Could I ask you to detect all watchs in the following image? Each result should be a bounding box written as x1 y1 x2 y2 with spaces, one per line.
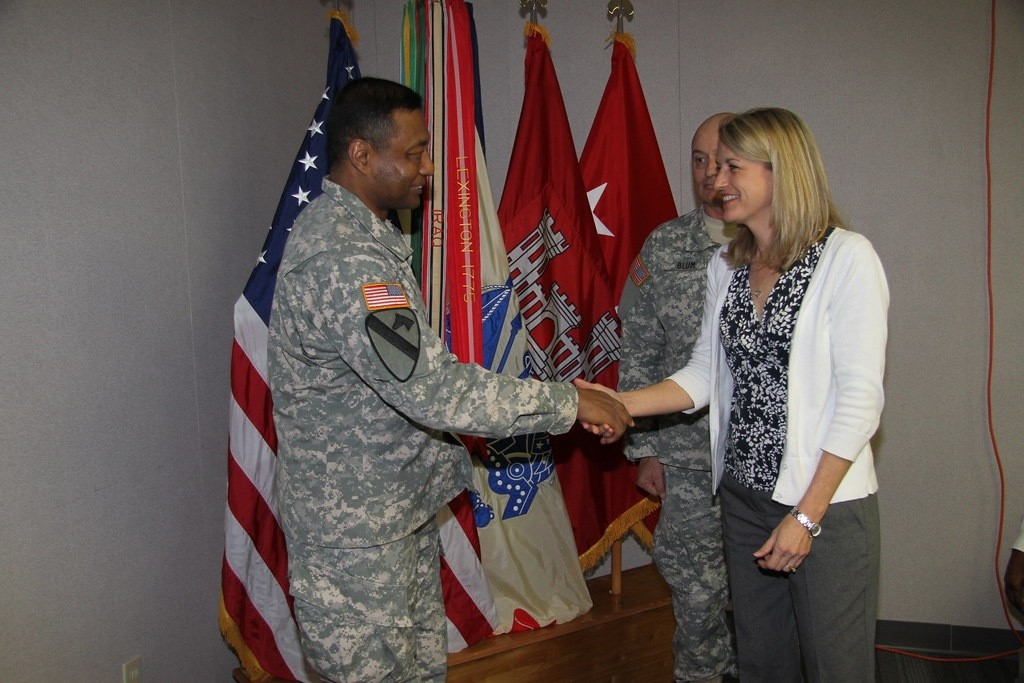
789 503 823 539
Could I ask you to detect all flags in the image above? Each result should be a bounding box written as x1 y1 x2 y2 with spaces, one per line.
215 0 494 683
402 0 592 633
491 19 682 571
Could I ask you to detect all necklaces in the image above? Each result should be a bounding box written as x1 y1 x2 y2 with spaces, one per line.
750 263 778 297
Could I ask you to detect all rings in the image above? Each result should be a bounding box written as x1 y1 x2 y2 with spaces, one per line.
786 562 796 572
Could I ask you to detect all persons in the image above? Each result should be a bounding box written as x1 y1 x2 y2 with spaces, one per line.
569 108 891 683
618 110 738 683
269 74 643 683
